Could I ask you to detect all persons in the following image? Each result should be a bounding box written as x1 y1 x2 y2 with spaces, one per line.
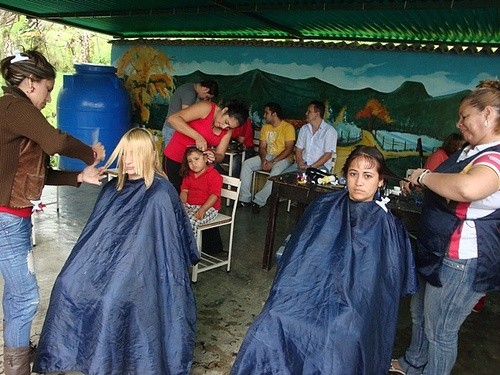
32 128 203 375
236 102 296 207
386 80 500 375
0 49 109 375
280 100 336 175
226 145 417 375
160 80 253 260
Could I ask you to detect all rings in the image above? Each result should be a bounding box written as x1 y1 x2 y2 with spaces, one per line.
401 185 405 189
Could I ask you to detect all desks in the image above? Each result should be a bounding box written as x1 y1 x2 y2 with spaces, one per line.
263 171 423 272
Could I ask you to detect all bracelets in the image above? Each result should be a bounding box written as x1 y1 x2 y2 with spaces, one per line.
418 168 431 188
271 159 275 164
92 148 98 161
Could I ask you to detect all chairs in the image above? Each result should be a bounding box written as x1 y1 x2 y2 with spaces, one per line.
189 150 292 283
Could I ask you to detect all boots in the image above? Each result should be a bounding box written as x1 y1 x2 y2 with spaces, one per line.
3 345 30 375
30 340 37 362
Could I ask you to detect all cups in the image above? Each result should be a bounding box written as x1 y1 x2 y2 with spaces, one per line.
394 186 401 196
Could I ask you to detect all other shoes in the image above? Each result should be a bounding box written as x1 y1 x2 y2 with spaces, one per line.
253 203 260 215
388 359 407 375
237 201 250 208
